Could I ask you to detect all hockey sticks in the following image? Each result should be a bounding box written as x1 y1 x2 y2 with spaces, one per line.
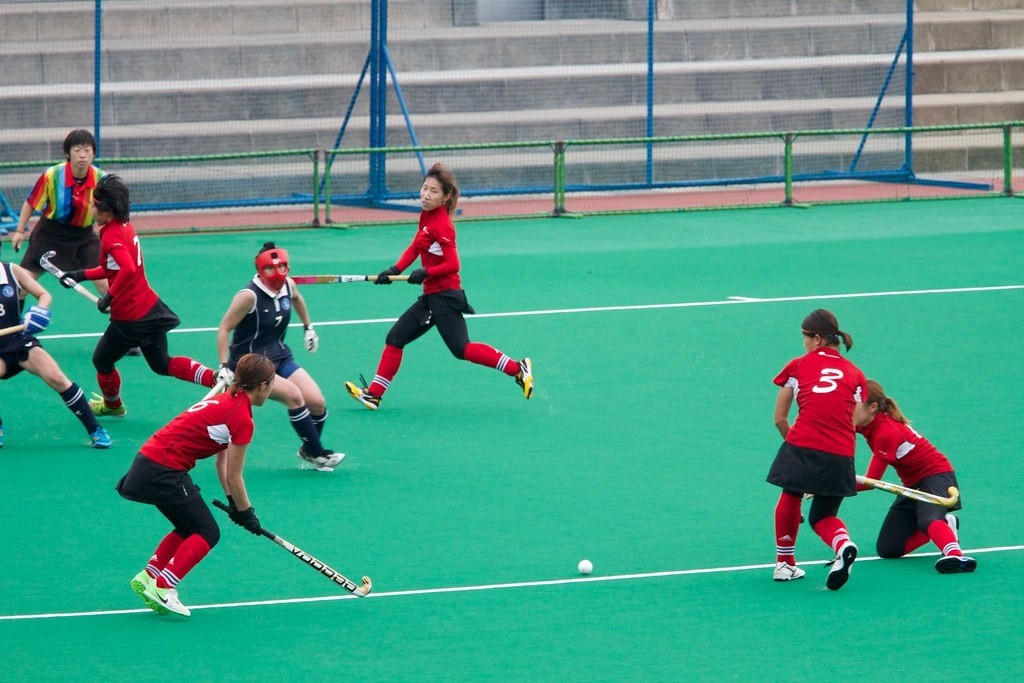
856 474 961 508
0 324 26 337
211 497 373 599
291 274 412 285
38 250 112 312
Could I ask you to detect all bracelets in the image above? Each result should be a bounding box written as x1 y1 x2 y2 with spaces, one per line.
16 230 25 234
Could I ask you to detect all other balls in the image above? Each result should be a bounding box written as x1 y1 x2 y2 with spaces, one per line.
578 559 593 574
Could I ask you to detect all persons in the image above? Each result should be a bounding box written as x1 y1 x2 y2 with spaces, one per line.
344 162 533 411
12 129 141 356
217 241 346 472
115 353 275 617
852 379 977 574
766 308 868 591
0 241 113 450
60 174 220 418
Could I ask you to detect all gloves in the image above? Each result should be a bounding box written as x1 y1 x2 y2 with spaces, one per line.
21 306 51 339
226 495 240 524
240 507 261 537
96 291 112 314
305 329 318 354
408 268 426 285
217 368 234 385
374 266 400 284
59 270 85 288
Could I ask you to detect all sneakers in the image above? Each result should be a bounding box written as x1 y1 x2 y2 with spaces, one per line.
89 391 126 418
127 347 141 356
1 426 4 446
345 374 380 411
942 514 960 543
311 452 346 467
89 426 113 448
773 561 805 581
515 358 533 398
214 367 228 395
296 447 316 463
131 570 170 616
143 579 191 617
826 542 858 591
935 556 977 574
